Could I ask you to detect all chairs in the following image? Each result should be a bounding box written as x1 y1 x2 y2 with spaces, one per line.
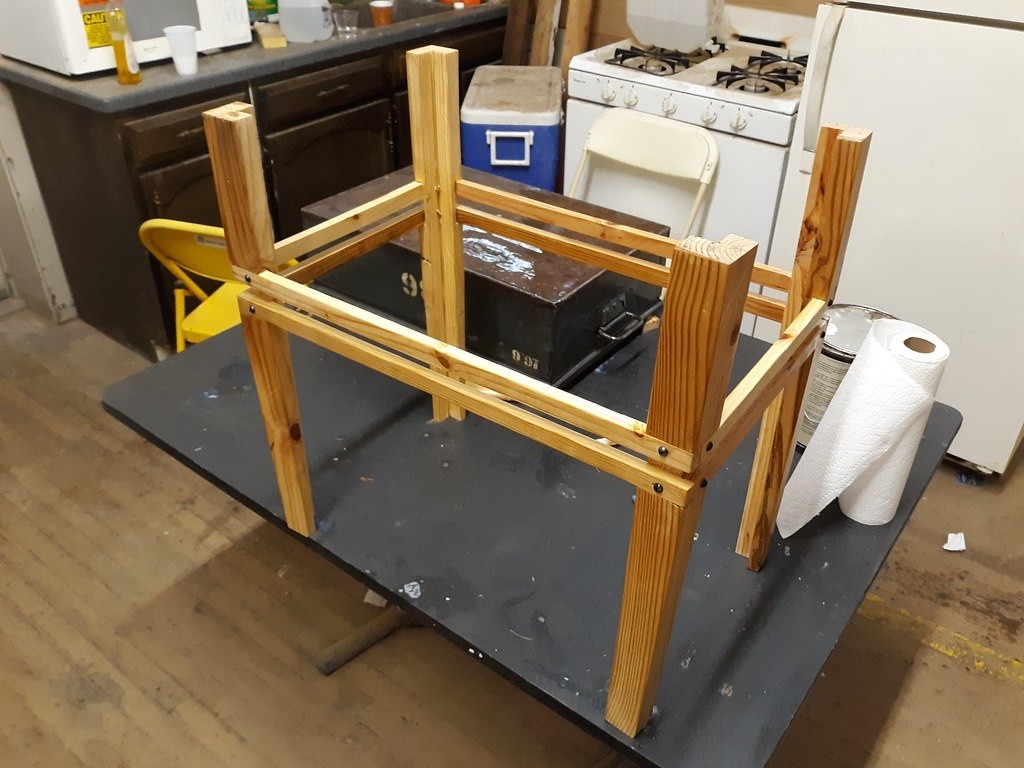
141 214 313 354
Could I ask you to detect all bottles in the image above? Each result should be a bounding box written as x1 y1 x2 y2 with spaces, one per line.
103 0 142 87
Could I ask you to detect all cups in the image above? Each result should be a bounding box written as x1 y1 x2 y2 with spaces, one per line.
331 10 359 39
368 0 394 28
163 25 197 76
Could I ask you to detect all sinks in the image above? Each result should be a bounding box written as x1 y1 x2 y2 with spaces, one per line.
325 0 458 34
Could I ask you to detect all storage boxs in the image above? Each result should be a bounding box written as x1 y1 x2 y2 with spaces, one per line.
459 61 566 197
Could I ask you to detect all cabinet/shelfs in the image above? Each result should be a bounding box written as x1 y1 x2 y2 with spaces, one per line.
0 0 513 366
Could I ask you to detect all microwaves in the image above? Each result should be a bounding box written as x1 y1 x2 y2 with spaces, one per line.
1 0 255 79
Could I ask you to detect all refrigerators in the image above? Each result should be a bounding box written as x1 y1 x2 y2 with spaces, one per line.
752 2 1024 486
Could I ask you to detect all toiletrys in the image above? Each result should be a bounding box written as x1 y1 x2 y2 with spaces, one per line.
103 1 145 86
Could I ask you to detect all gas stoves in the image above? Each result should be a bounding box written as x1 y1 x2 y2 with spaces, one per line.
604 36 808 98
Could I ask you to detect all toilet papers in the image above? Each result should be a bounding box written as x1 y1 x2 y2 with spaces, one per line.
773 316 951 542
